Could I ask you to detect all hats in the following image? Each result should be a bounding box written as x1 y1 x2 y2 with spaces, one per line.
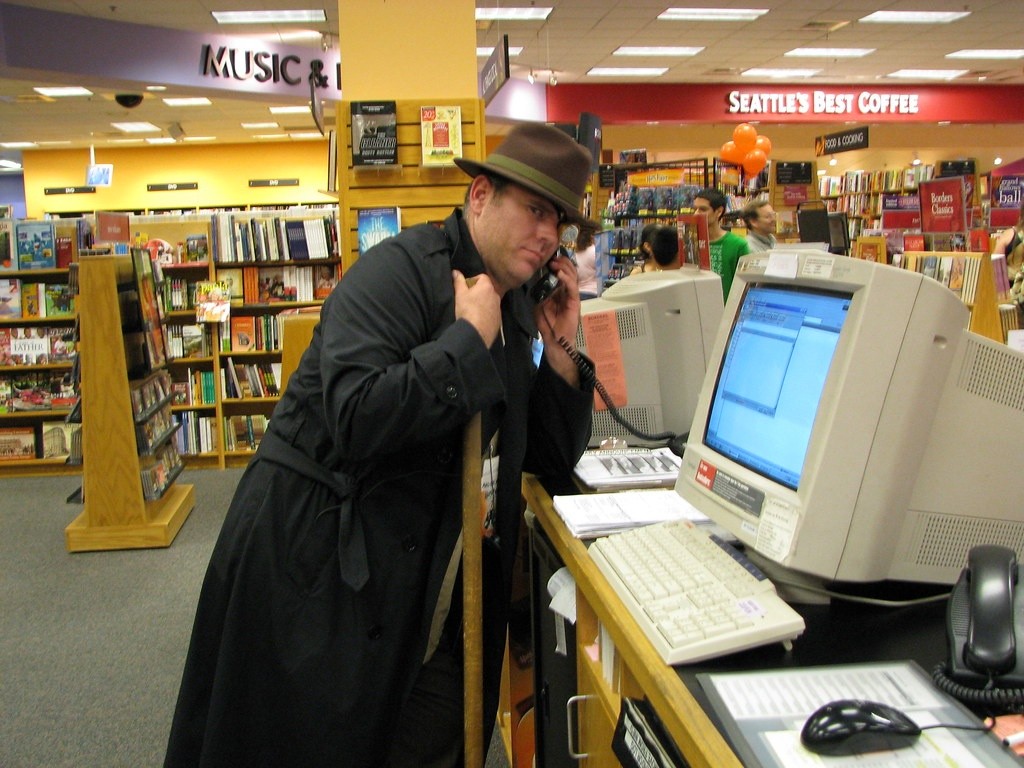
452 122 603 232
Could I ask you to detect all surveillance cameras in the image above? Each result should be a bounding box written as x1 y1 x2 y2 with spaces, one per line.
114 91 143 108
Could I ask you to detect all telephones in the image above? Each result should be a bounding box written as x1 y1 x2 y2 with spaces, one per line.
531 245 570 306
944 545 1024 684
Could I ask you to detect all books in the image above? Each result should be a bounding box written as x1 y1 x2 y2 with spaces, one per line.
211 203 336 263
0 277 75 318
0 369 78 413
171 359 285 405
168 309 285 357
132 370 180 499
173 412 272 456
0 420 83 466
0 327 75 365
165 263 344 310
818 164 1018 346
0 203 132 273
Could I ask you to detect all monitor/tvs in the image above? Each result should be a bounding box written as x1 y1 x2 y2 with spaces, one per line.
673 249 1024 605
565 264 725 447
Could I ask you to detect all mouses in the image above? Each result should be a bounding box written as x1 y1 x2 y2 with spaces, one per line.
801 699 921 757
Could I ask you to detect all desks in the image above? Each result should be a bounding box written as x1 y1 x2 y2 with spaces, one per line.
492 445 1024 768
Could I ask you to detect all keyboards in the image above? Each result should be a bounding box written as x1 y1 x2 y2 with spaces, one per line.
588 514 806 668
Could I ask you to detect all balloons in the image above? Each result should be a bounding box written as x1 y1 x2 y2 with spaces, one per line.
721 124 771 178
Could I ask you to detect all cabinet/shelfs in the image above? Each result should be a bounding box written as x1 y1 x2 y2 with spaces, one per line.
1 186 1024 554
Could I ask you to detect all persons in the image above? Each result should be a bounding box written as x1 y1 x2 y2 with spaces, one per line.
993 195 1024 319
54 335 67 355
15 355 21 364
631 221 685 276
571 222 600 300
11 327 18 338
37 328 45 338
162 119 597 768
38 354 48 363
24 328 31 338
690 187 749 304
740 200 779 255
